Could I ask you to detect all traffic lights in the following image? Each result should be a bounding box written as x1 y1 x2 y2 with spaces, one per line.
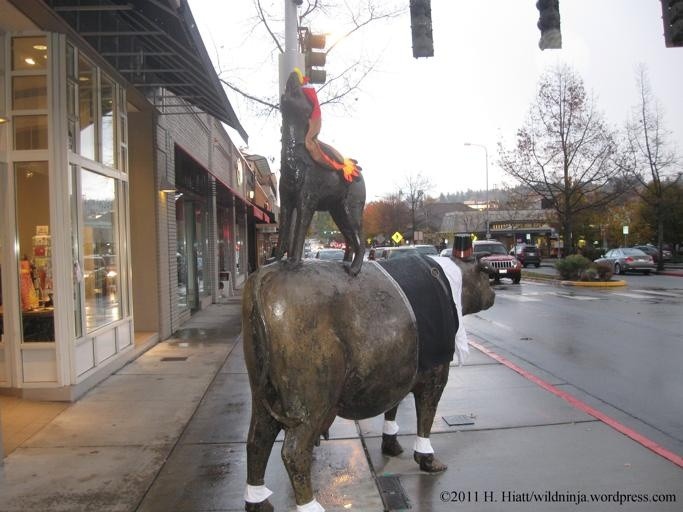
297 29 327 87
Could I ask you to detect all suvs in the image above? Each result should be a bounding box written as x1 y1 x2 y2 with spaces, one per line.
471 240 521 285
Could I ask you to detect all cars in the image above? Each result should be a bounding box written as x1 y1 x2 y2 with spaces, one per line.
510 244 541 267
591 244 672 273
306 246 452 268
84 254 119 297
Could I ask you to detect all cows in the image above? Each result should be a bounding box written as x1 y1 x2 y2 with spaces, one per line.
241 248 497 512
